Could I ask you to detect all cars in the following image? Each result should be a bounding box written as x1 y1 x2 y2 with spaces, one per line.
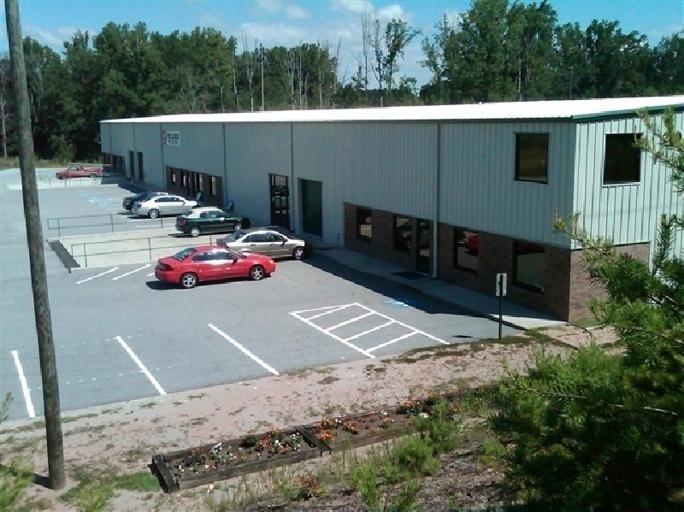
131 195 197 219
465 231 546 254
123 190 169 214
216 228 309 261
155 246 275 288
175 208 251 237
396 219 466 249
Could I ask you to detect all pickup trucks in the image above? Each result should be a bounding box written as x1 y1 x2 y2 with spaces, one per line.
56 164 101 179
